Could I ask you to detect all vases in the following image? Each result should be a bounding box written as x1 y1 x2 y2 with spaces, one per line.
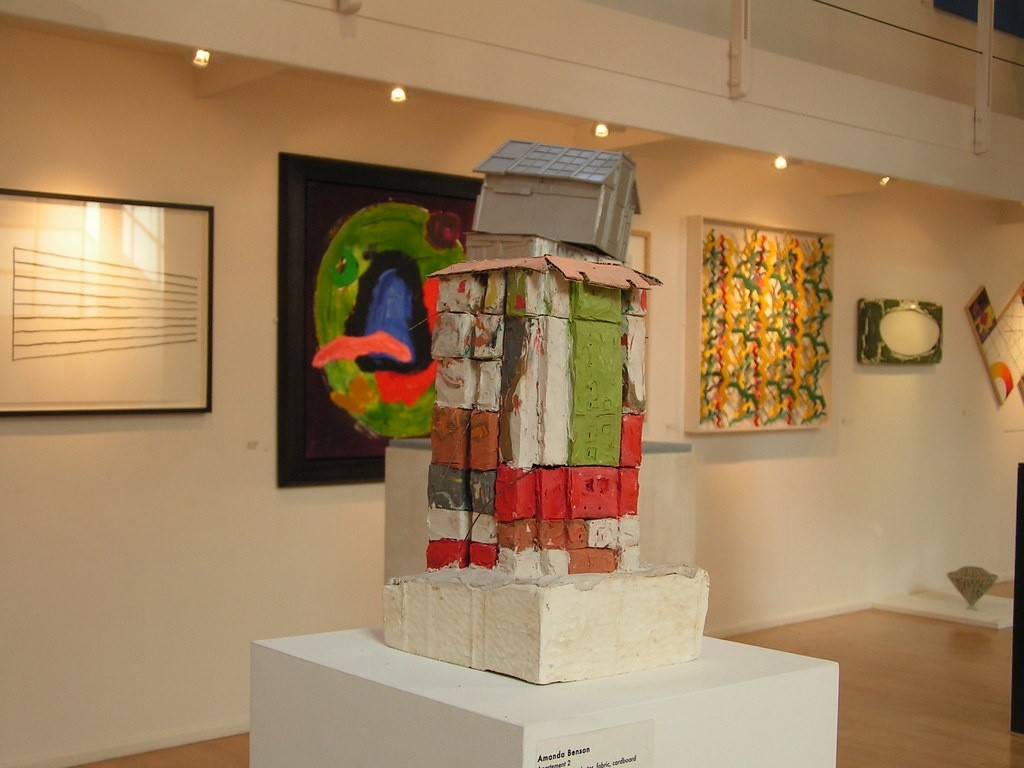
274 151 484 488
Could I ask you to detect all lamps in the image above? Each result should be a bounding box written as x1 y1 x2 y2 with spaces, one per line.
858 292 943 366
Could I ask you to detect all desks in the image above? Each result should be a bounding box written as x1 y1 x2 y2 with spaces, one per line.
247 628 841 768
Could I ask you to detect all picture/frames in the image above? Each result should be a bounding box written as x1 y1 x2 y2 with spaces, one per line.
686 211 834 441
625 231 651 278
965 280 1024 404
2 187 216 417
